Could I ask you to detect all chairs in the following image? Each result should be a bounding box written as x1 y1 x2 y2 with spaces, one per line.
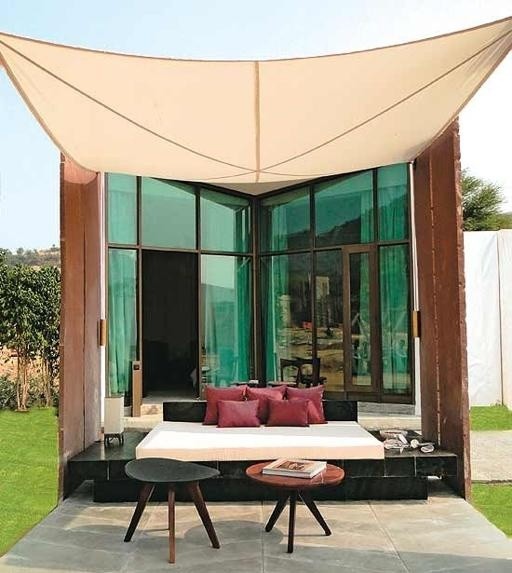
280 356 327 385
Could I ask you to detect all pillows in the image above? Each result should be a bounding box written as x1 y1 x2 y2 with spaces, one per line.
202 384 328 428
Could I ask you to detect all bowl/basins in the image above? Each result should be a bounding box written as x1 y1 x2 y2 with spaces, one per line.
379 429 408 440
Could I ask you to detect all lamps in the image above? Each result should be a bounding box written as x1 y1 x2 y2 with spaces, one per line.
103 395 126 448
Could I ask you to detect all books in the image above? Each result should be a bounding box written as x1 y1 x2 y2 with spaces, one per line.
261 457 328 480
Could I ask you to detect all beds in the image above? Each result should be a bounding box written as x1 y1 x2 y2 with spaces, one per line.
134 400 385 462
